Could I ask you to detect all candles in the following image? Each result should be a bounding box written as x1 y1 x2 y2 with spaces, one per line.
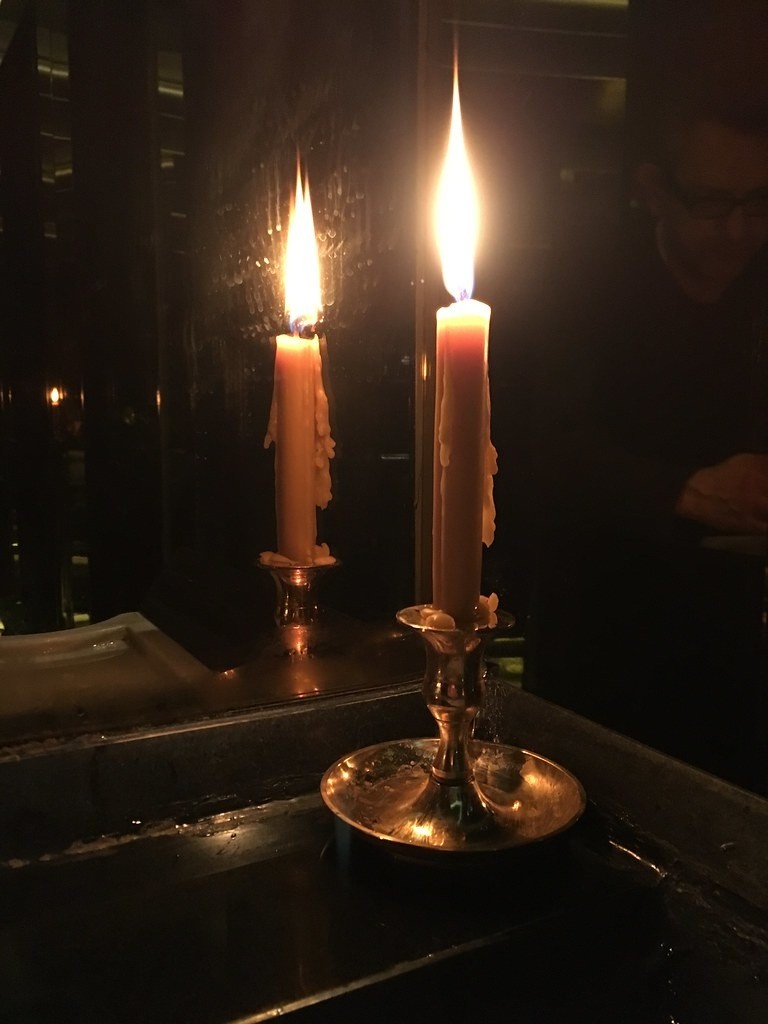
430 37 500 614
264 139 337 560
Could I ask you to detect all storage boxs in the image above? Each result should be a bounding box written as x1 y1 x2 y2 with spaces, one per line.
0 676 768 1024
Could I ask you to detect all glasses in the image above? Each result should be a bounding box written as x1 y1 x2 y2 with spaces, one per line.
654 159 768 222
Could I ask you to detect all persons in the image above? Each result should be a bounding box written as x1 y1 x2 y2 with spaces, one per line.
530 80 768 791
